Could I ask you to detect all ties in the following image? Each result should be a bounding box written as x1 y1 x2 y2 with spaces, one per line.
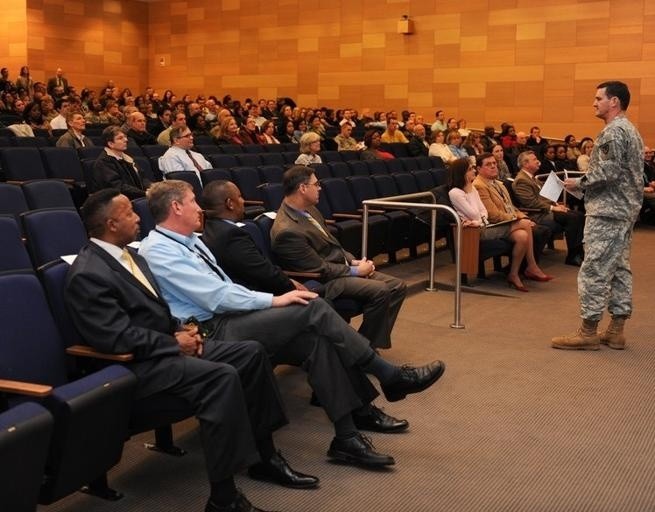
122 251 158 298
186 149 203 172
307 213 329 237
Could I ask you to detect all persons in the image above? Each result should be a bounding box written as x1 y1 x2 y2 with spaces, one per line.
3 63 591 193
63 165 444 512
448 150 592 293
643 144 655 207
551 80 645 352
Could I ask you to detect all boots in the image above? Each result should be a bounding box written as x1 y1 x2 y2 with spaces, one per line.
551 319 600 350
598 317 625 349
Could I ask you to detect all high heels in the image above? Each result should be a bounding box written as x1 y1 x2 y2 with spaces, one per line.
524 270 554 281
507 275 528 292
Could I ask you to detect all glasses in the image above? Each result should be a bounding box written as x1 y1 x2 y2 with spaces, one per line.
306 181 320 186
176 133 193 138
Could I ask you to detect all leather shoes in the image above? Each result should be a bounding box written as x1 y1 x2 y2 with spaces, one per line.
327 432 394 465
355 402 409 432
247 449 319 488
204 488 264 512
380 360 445 402
565 256 583 266
